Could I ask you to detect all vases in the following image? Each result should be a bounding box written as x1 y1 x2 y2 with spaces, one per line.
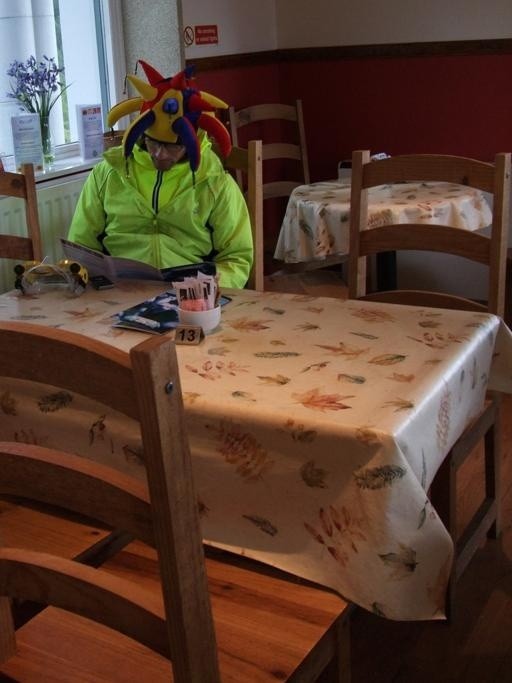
36 111 55 169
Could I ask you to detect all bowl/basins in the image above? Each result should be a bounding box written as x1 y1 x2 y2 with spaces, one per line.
177 305 222 334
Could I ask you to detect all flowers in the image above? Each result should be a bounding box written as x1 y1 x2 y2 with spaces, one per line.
7 54 78 153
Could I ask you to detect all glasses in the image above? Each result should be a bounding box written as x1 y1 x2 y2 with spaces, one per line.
142 139 183 153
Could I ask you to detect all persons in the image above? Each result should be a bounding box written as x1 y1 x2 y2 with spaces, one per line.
64 59 253 288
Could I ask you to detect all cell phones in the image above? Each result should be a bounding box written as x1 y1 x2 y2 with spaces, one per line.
89 274 114 291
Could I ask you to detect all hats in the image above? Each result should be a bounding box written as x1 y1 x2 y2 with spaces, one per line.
106 59 232 189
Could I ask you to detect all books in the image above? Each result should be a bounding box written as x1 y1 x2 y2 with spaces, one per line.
57 236 218 281
107 285 234 336
